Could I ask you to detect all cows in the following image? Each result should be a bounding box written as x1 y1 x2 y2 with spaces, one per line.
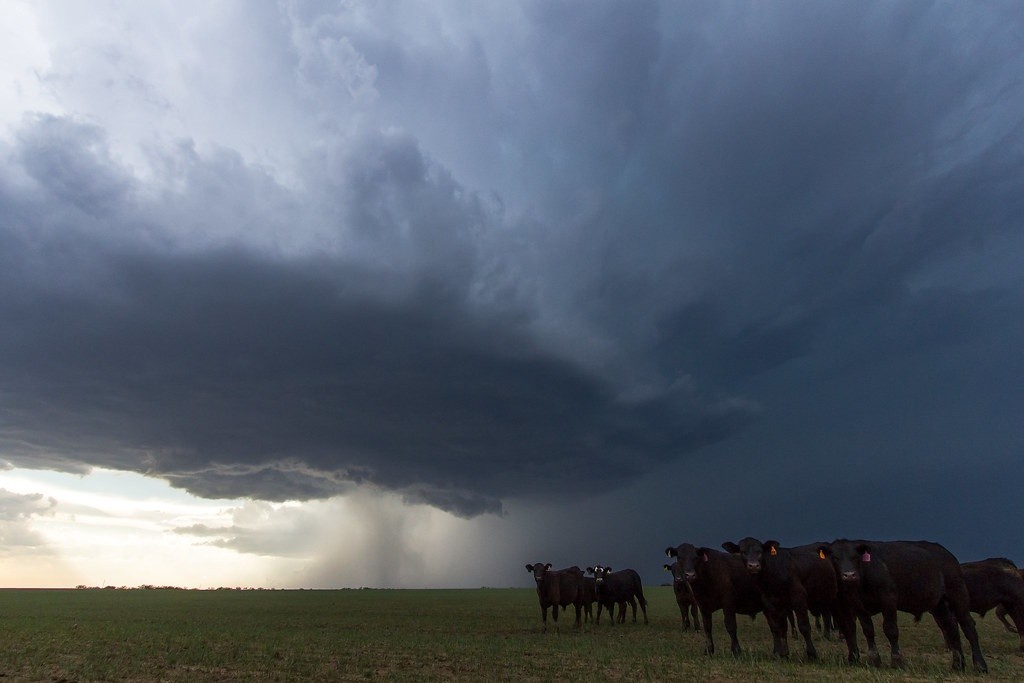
660 537 1024 674
524 562 651 628
